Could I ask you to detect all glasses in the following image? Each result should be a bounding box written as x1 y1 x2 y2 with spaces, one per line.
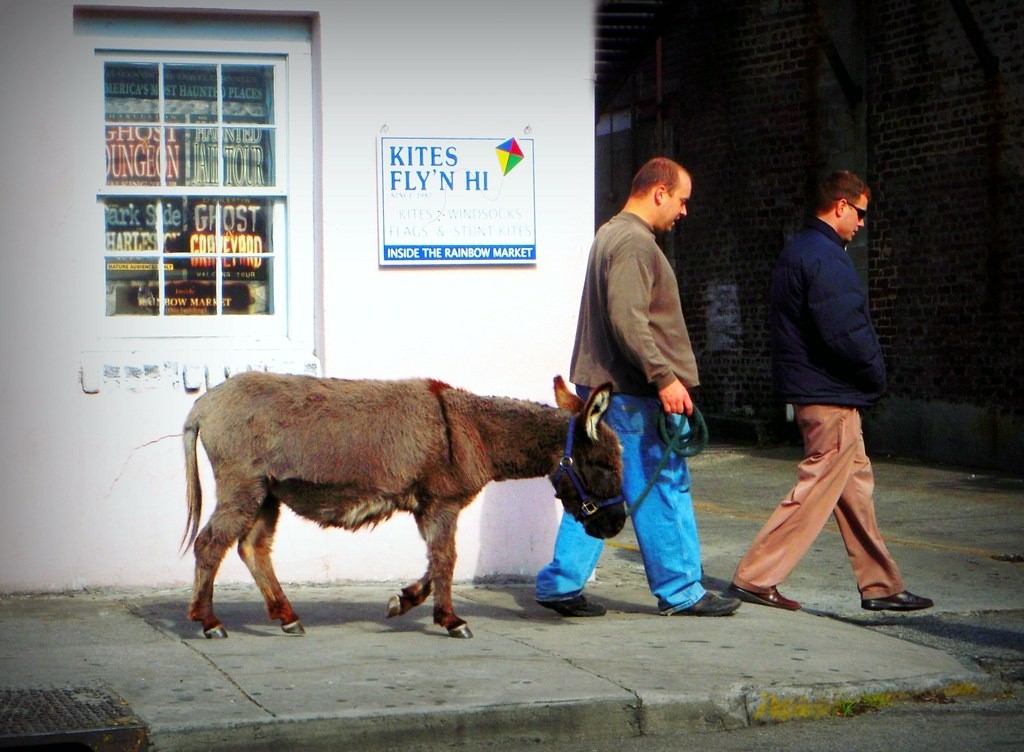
836 196 867 219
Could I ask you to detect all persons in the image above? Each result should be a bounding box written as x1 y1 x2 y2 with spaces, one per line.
534 155 742 619
727 168 936 613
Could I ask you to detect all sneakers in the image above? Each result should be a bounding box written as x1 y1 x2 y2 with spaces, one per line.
536 593 607 617
674 591 742 615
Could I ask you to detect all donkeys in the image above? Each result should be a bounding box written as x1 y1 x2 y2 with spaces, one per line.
175 371 624 641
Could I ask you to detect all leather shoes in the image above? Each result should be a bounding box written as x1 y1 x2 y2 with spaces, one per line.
859 589 934 611
728 580 802 611
